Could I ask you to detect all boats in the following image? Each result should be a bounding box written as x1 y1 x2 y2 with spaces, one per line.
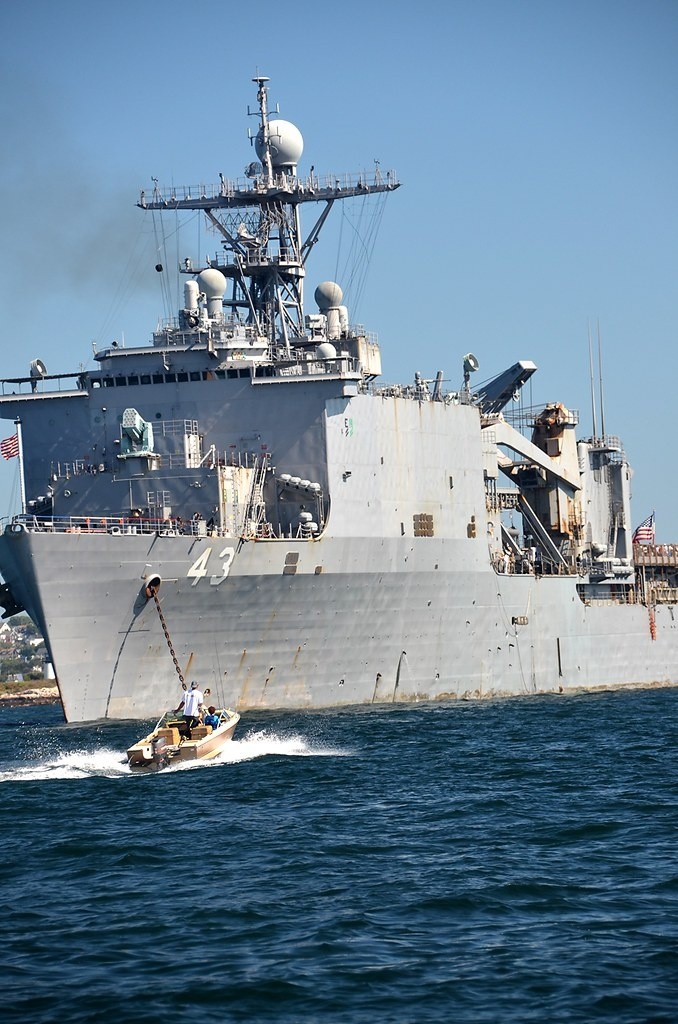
126 702 242 774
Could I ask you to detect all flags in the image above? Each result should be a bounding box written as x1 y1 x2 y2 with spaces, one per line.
629 512 656 548
0 432 21 463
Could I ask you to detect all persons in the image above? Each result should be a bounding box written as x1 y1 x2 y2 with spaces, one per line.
628 571 675 603
201 705 223 729
503 541 511 573
576 553 583 574
172 679 206 744
128 507 218 536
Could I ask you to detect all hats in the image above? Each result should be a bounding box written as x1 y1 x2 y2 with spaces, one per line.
191 680 199 688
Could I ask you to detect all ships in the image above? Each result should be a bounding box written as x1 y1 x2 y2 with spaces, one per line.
1 69 676 719
